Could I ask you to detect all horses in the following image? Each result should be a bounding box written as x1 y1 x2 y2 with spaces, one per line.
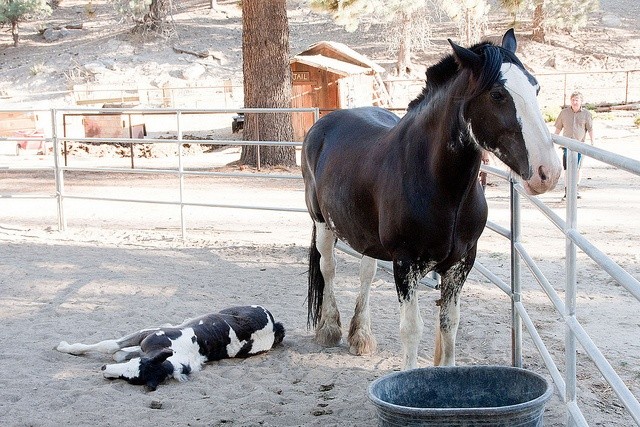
300 27 562 373
57 305 286 394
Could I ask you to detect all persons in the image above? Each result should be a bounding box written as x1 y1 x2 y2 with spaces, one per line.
553 91 594 200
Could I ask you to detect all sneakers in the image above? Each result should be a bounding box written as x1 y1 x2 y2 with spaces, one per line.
562 194 566 201
577 191 581 199
